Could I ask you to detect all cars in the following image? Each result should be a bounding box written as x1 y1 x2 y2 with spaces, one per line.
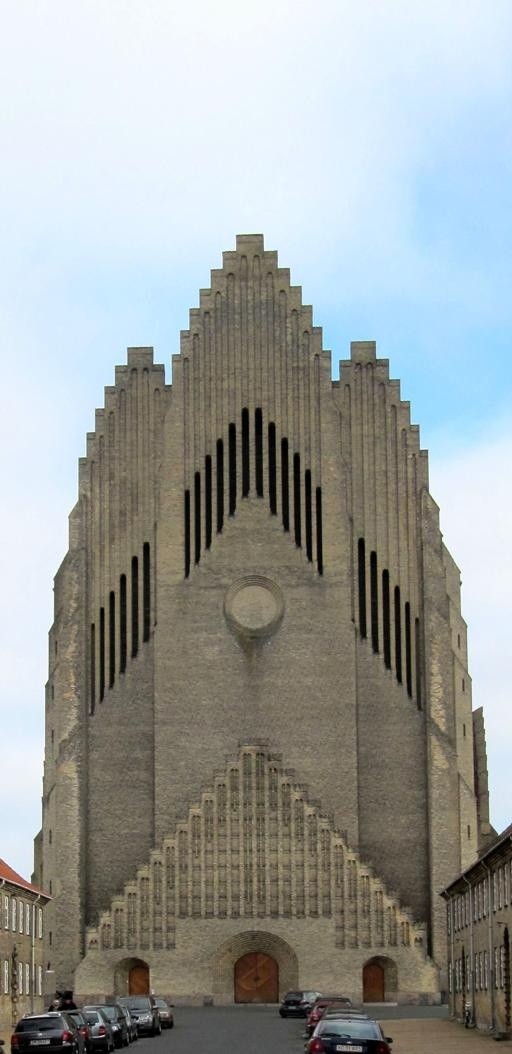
11 995 174 1054
280 990 393 1054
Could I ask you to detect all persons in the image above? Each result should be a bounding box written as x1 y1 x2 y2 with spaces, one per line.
48 996 77 1011
464 1008 471 1028
